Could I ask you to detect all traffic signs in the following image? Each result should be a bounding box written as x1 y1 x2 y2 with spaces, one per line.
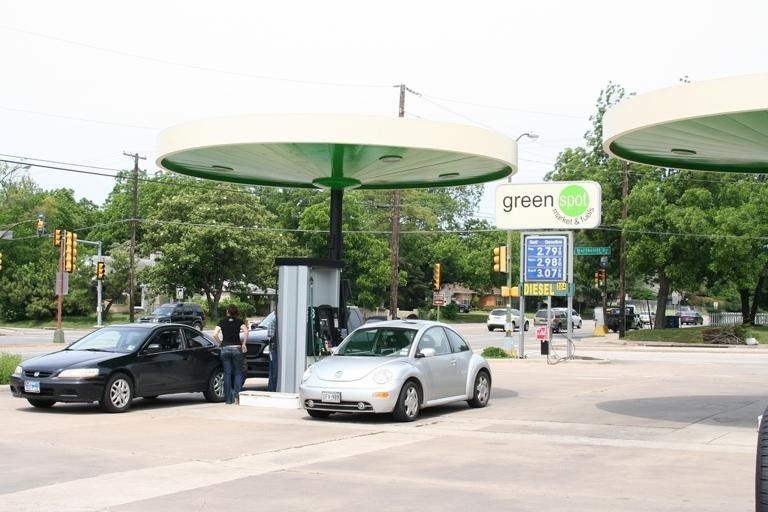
574 246 611 255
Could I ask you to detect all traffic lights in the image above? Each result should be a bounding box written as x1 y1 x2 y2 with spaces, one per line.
38 220 46 232
98 262 105 279
54 230 62 246
599 268 607 287
500 285 509 297
433 263 441 291
64 231 77 272
493 245 507 273
595 273 599 288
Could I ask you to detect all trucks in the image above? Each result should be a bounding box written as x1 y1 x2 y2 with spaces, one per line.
600 304 644 331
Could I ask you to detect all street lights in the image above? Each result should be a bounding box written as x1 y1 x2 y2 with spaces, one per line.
0 164 32 182
504 130 539 338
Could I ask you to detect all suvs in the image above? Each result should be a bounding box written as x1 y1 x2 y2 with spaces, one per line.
139 303 205 330
236 304 365 379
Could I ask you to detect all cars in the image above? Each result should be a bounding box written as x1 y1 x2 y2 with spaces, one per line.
680 312 703 325
639 311 656 324
553 306 584 330
486 308 530 332
676 306 694 316
451 298 470 313
299 319 494 420
9 321 249 412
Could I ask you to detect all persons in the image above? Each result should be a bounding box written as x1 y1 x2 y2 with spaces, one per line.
213 304 248 403
266 307 277 392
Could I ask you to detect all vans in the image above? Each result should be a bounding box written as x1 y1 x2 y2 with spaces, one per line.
534 309 574 332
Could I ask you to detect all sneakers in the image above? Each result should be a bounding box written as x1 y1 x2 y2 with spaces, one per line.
234 395 239 404
